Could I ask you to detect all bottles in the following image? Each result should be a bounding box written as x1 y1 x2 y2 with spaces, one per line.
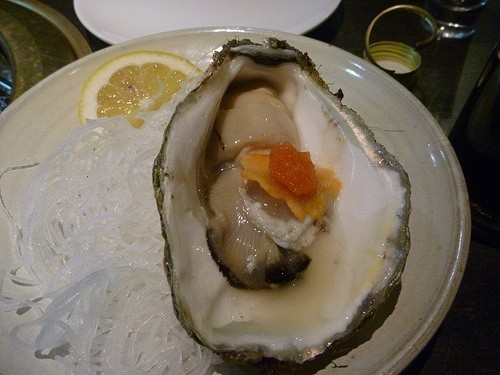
445 37 499 248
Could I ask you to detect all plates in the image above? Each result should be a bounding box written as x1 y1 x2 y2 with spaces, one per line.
72 0 342 47
1 26 472 374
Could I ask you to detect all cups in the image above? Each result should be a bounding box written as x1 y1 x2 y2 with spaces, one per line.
428 0 487 50
362 5 438 93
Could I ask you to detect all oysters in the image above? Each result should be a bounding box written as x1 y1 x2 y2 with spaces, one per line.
153 39 414 368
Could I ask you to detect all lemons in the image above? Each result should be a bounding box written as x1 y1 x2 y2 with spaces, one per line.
77 50 205 133
